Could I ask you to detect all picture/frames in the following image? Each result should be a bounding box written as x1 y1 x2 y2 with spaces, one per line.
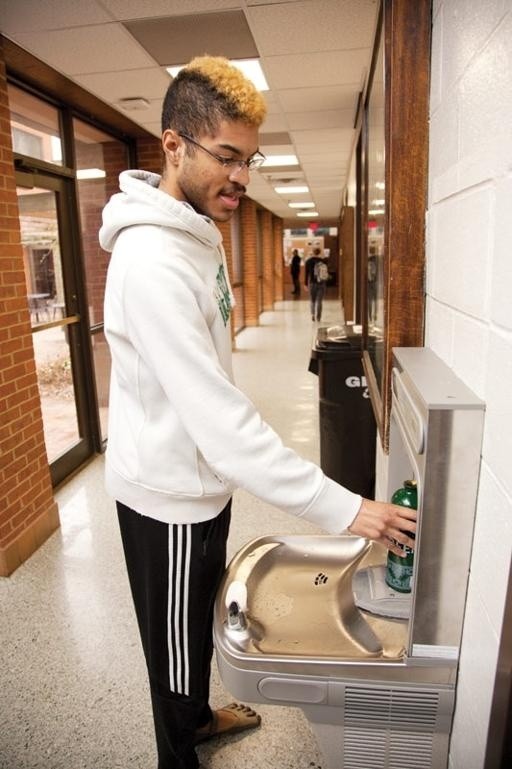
361 0 433 456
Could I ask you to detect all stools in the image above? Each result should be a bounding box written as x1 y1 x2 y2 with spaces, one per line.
53 304 64 320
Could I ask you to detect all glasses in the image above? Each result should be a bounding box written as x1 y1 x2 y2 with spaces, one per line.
177 132 266 175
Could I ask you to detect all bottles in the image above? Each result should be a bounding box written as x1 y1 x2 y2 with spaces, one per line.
385 479 419 592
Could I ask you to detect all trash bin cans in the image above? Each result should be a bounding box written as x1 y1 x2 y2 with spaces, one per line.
312 336 378 501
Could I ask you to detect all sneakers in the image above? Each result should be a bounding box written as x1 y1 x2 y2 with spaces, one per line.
312 316 320 322
193 702 261 746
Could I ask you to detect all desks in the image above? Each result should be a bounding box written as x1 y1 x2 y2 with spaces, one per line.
28 293 50 323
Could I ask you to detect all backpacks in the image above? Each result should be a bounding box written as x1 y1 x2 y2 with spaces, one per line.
314 261 332 282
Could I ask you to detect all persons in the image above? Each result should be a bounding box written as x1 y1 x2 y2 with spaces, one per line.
367 246 378 322
98 52 418 769
289 249 302 298
303 248 333 322
